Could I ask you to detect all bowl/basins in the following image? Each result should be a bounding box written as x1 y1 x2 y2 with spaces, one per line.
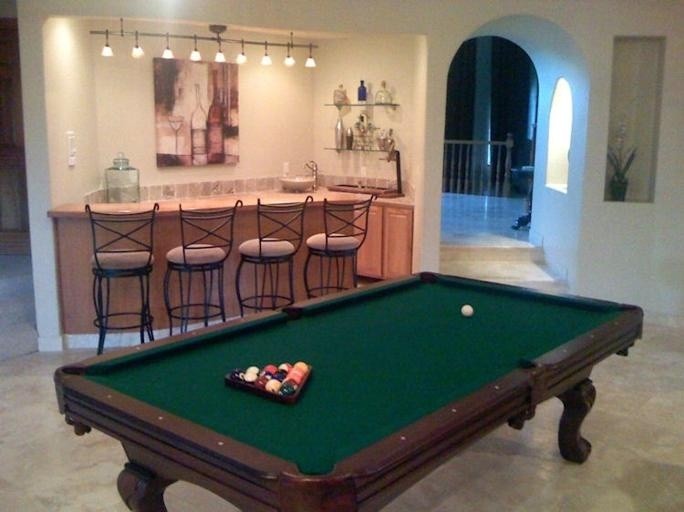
280 177 316 191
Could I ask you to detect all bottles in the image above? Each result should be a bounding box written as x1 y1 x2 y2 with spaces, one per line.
191 85 208 165
335 116 344 150
104 154 140 204
334 82 347 104
208 69 225 163
374 80 392 105
347 127 354 149
358 80 367 104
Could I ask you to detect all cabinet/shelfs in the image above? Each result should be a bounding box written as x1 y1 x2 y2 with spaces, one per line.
354 201 382 280
382 202 412 281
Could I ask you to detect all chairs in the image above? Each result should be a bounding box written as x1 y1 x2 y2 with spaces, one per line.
304 194 378 299
235 196 313 317
85 202 159 356
163 200 243 336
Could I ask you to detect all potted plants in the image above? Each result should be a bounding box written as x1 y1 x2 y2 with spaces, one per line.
607 123 639 202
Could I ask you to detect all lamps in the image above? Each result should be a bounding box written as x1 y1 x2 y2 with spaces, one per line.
215 36 225 62
237 39 247 64
262 41 271 66
190 34 202 61
131 31 143 58
101 28 113 57
305 42 316 68
162 32 174 59
284 42 295 66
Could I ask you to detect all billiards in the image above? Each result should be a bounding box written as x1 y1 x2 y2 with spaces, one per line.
265 379 282 393
258 370 273 380
282 378 298 391
278 384 295 396
461 304 476 318
278 363 293 371
263 364 278 375
230 368 246 382
275 369 288 377
254 377 269 390
291 367 305 378
294 361 308 373
287 373 301 384
244 371 258 382
246 366 259 374
